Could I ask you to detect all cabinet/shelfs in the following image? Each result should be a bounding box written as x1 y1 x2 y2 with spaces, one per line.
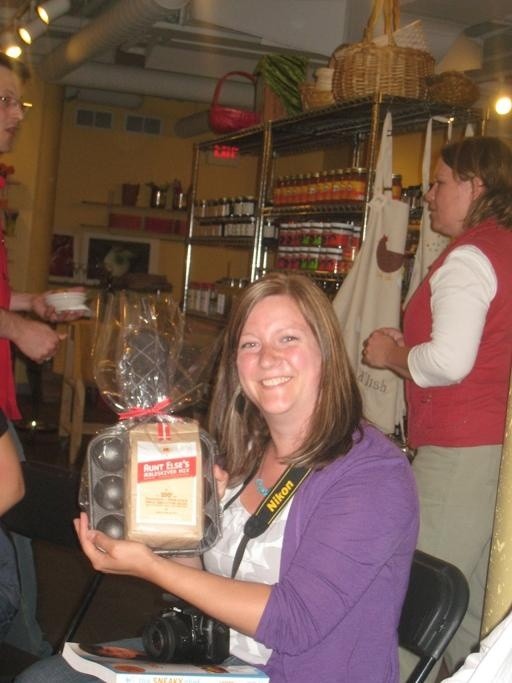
171 94 488 421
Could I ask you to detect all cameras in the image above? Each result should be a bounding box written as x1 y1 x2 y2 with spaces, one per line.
142 603 230 666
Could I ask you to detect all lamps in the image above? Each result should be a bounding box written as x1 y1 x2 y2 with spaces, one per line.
14 1 70 45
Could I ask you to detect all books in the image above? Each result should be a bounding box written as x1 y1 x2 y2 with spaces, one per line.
61 633 270 683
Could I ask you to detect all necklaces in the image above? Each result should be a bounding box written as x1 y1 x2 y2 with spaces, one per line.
249 449 277 501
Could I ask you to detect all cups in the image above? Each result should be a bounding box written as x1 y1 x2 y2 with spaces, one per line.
121 183 139 207
150 189 168 209
172 192 188 210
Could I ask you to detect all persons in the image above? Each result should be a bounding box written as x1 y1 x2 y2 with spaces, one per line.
1 406 28 521
6 268 420 683
358 133 509 683
2 47 89 464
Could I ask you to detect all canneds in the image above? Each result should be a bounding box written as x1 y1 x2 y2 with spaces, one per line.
193 196 278 240
273 169 403 275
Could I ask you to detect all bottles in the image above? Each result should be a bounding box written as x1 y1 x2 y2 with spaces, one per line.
187 274 250 322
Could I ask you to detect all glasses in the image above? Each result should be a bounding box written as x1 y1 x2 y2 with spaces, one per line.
0 97 31 112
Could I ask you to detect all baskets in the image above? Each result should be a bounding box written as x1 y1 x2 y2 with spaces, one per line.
330 0 435 105
209 70 262 135
296 70 480 111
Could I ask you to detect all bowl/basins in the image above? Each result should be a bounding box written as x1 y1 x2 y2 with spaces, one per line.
45 285 91 314
